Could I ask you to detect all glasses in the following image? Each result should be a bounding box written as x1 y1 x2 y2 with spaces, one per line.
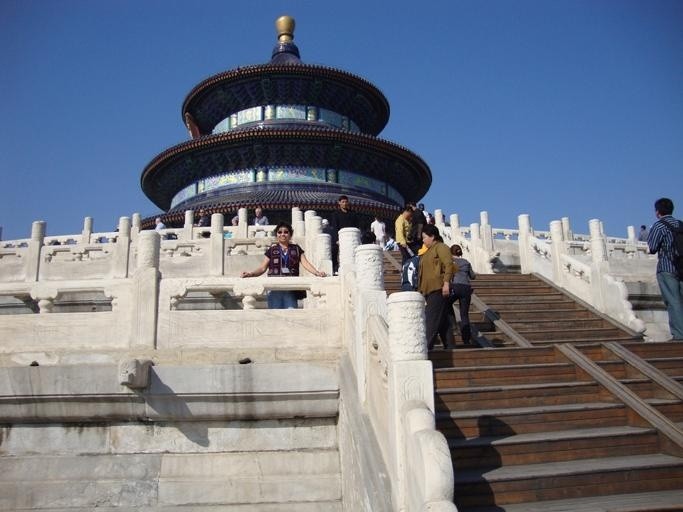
278 230 288 234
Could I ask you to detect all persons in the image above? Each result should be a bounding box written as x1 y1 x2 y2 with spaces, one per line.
193 208 209 238
360 203 444 293
240 221 327 310
329 196 358 277
446 243 475 345
415 223 456 352
153 217 168 240
250 208 269 225
645 197 683 343
636 224 649 242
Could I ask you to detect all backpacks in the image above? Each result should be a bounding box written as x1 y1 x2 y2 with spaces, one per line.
658 218 683 274
401 255 420 290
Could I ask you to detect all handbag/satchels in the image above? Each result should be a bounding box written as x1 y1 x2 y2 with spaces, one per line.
292 290 307 300
